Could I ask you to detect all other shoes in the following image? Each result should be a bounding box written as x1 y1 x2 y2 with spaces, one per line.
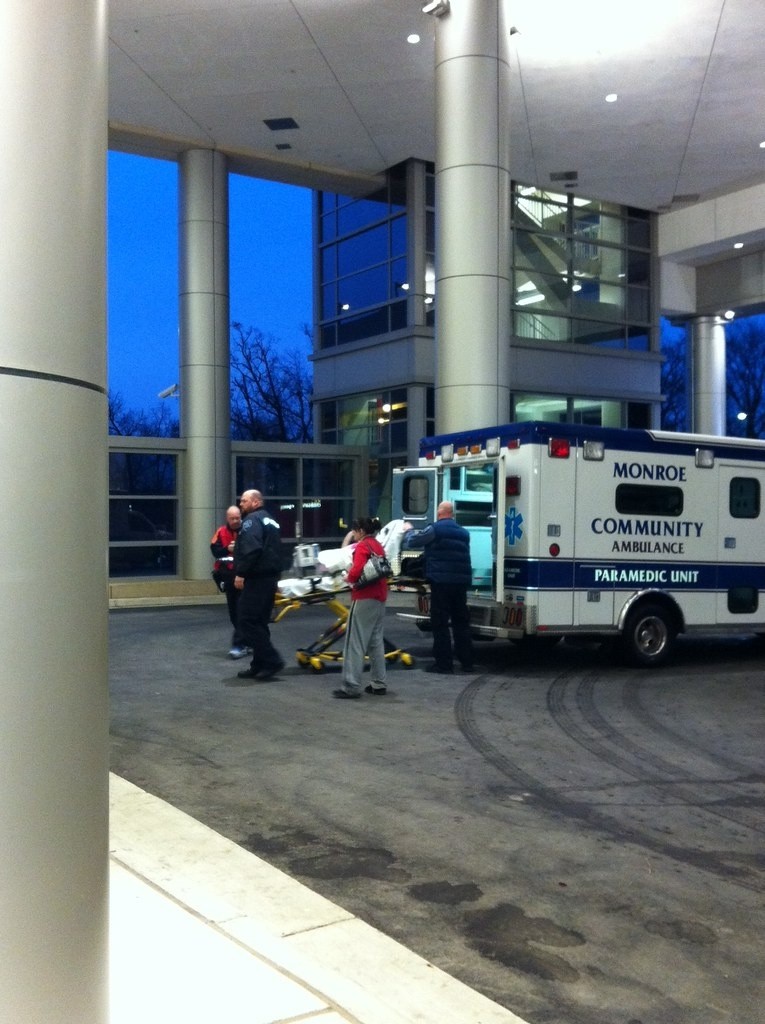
237 644 285 681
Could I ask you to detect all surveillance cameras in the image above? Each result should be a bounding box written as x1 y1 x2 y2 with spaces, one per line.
158 384 177 398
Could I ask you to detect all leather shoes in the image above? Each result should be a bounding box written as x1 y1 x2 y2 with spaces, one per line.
461 662 473 672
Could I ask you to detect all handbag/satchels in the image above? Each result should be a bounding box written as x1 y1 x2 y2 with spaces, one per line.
210 569 226 593
352 540 394 590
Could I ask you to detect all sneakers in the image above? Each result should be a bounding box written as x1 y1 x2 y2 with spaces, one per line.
426 663 454 675
364 684 387 696
332 689 362 699
227 644 254 660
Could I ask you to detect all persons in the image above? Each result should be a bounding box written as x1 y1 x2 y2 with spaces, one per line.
231 490 285 679
404 502 481 672
210 506 253 657
330 517 388 696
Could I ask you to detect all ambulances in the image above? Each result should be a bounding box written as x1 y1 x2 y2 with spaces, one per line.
391 420 765 666
